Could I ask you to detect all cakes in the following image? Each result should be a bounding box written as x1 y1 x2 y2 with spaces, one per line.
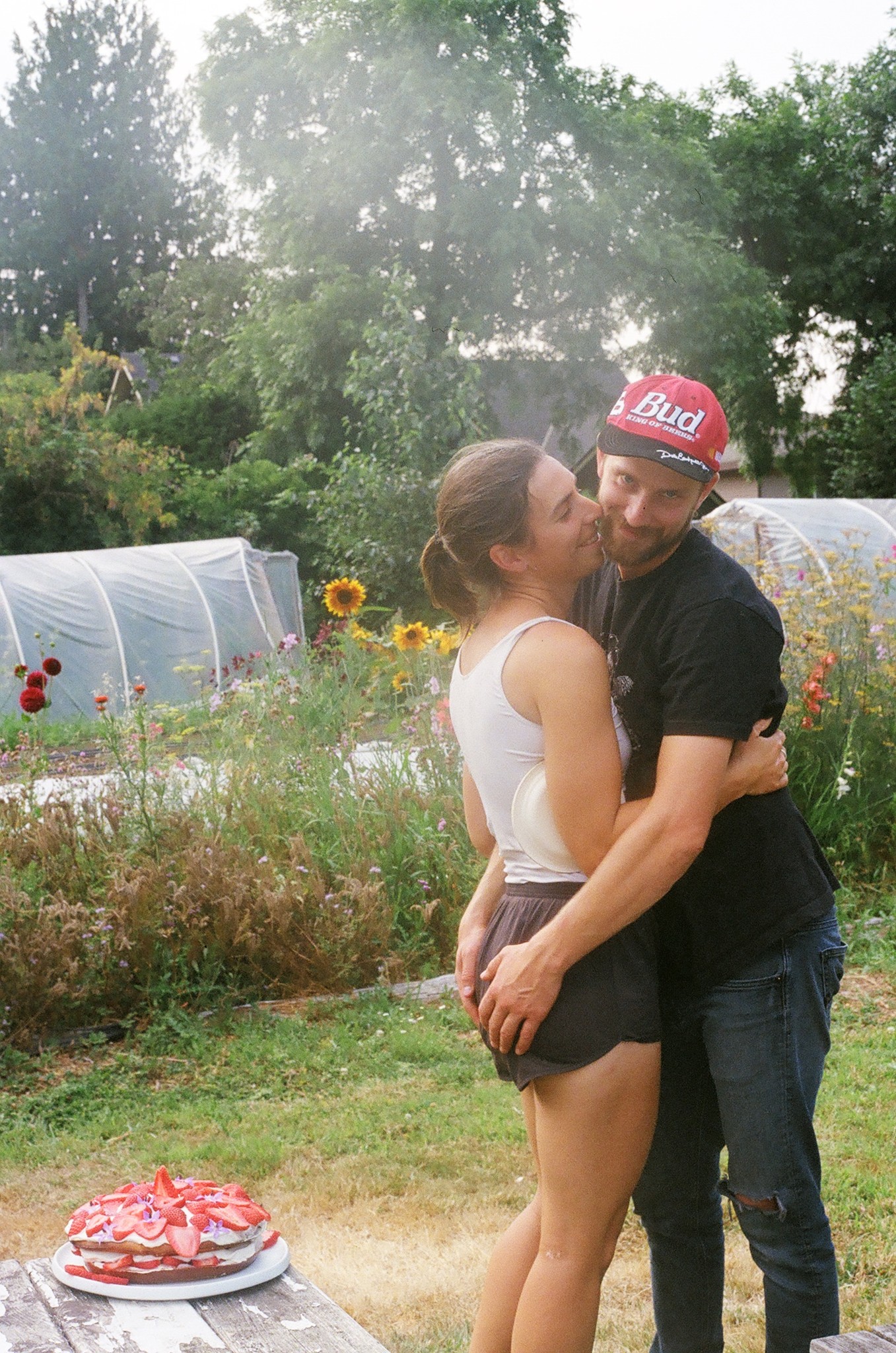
63 1166 281 1283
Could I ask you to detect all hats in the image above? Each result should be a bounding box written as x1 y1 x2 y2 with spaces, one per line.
598 375 728 482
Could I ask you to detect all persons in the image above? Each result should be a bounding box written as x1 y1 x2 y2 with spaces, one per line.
457 375 849 1353
419 439 790 1353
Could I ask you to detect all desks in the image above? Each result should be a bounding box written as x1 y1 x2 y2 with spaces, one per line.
0 1256 391 1353
